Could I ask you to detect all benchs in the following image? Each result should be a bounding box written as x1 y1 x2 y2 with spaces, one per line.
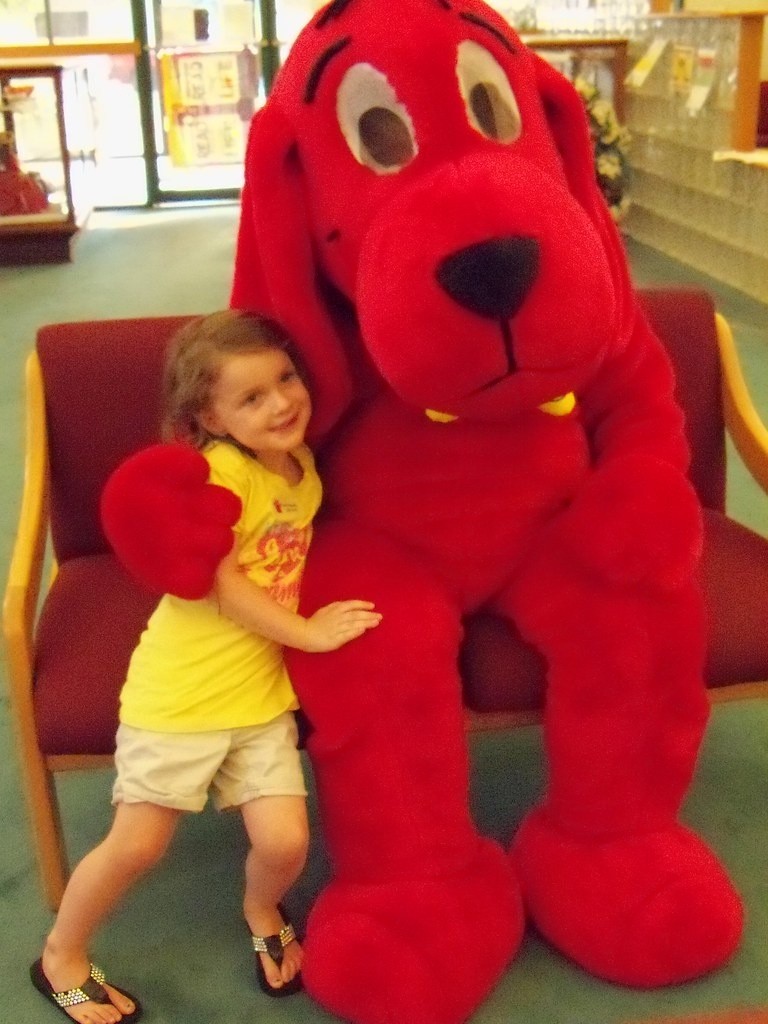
0 286 767 912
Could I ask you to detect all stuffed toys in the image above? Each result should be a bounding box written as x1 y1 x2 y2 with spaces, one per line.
100 2 750 1024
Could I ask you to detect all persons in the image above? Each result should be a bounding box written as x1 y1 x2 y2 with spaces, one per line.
30 302 384 1024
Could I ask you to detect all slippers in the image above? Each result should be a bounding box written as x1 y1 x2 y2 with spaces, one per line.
30 956 144 1024
252 899 307 997
